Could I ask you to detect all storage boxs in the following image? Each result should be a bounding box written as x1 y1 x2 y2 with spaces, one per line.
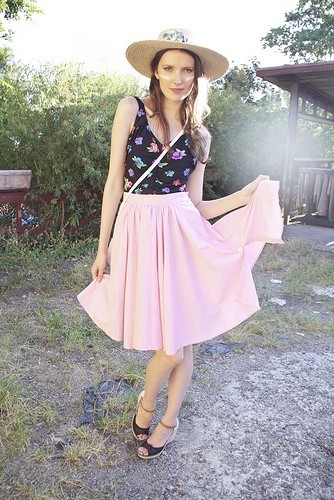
0 169 31 191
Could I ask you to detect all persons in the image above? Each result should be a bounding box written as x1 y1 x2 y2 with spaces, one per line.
76 27 286 460
